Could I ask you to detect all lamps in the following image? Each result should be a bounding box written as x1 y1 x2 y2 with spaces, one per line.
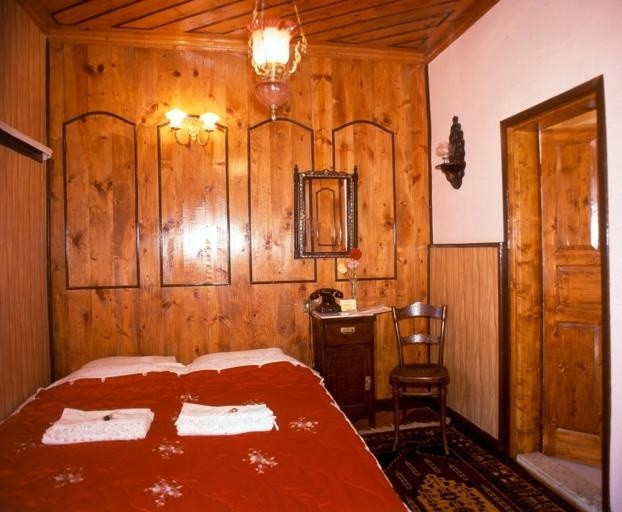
166 108 220 148
434 114 466 191
246 0 309 122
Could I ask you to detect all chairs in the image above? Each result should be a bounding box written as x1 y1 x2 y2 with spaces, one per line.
389 302 449 455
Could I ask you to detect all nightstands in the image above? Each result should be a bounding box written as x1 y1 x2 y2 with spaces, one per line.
309 310 377 429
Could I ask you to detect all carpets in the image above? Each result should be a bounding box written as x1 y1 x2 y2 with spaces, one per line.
356 415 585 511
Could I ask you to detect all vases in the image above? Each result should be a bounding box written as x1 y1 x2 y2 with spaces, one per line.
350 278 362 312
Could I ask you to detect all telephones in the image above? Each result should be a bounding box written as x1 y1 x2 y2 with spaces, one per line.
309 288 343 314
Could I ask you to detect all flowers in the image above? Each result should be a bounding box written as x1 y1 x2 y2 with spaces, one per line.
336 248 362 298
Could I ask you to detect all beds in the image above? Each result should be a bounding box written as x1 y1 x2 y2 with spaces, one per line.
1 348 408 508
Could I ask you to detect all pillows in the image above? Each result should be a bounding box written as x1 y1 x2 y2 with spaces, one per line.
80 347 283 369
